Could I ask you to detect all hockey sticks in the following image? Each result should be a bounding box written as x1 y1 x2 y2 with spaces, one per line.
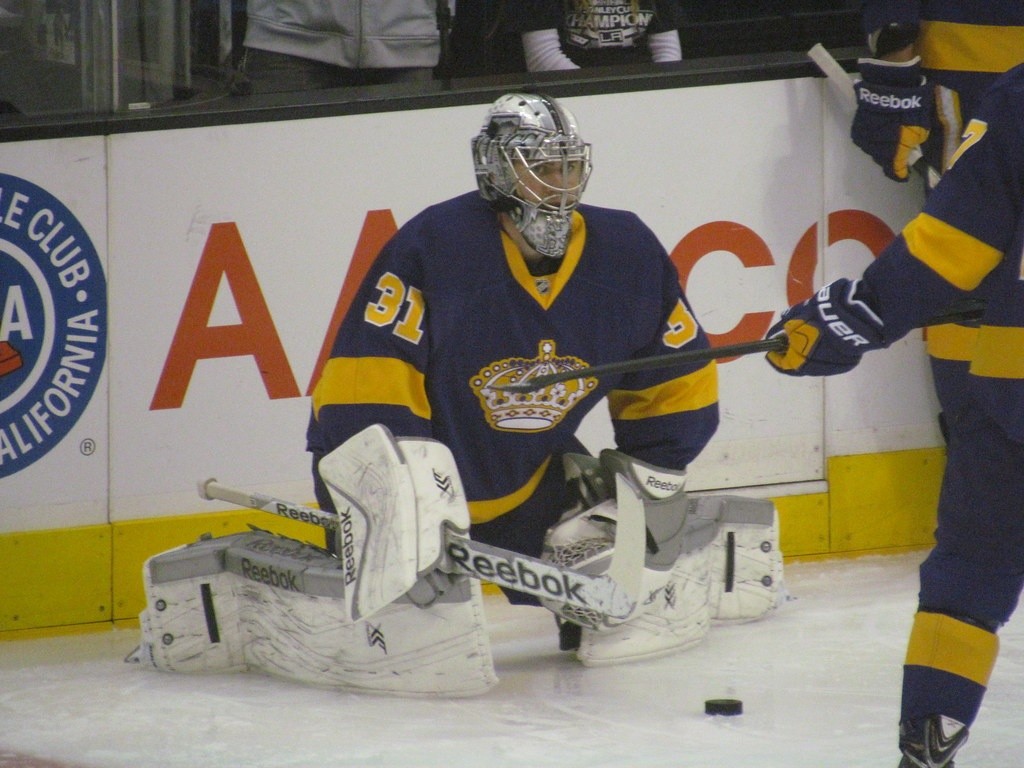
195 474 647 618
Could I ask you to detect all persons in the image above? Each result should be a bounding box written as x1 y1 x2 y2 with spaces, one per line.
241 0 455 90
766 73 1023 768
493 0 683 76
851 0 1024 195
139 91 788 700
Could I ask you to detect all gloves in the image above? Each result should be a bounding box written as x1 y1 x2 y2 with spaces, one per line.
764 272 893 377
542 436 688 634
851 56 936 183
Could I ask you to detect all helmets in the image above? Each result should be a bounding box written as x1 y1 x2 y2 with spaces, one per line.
473 91 592 259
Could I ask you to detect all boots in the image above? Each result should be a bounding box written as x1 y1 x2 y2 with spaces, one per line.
891 714 969 767
